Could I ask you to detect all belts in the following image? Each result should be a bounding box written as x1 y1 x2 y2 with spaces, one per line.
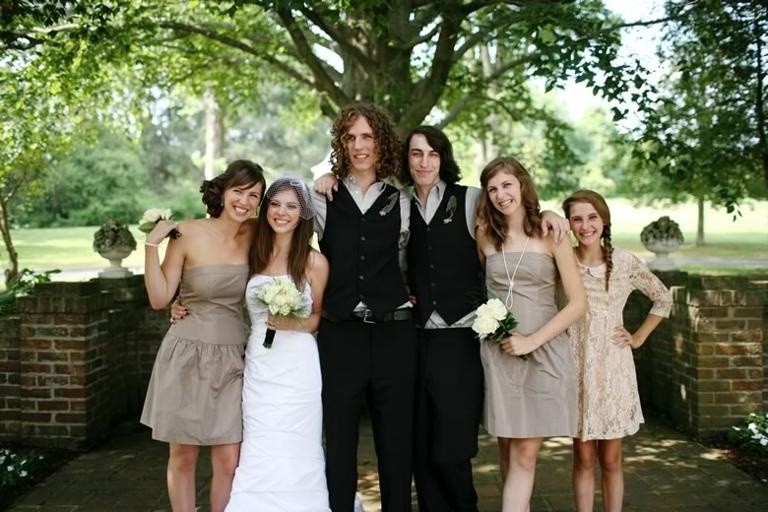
351 309 411 325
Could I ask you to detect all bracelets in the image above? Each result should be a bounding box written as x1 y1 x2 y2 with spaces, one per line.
144 241 160 248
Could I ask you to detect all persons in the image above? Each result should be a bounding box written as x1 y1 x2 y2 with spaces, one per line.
310 126 572 512
168 177 335 512
299 102 419 512
408 156 589 512
556 189 675 512
137 160 266 511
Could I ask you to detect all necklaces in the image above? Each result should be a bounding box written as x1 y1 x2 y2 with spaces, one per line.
498 221 538 310
